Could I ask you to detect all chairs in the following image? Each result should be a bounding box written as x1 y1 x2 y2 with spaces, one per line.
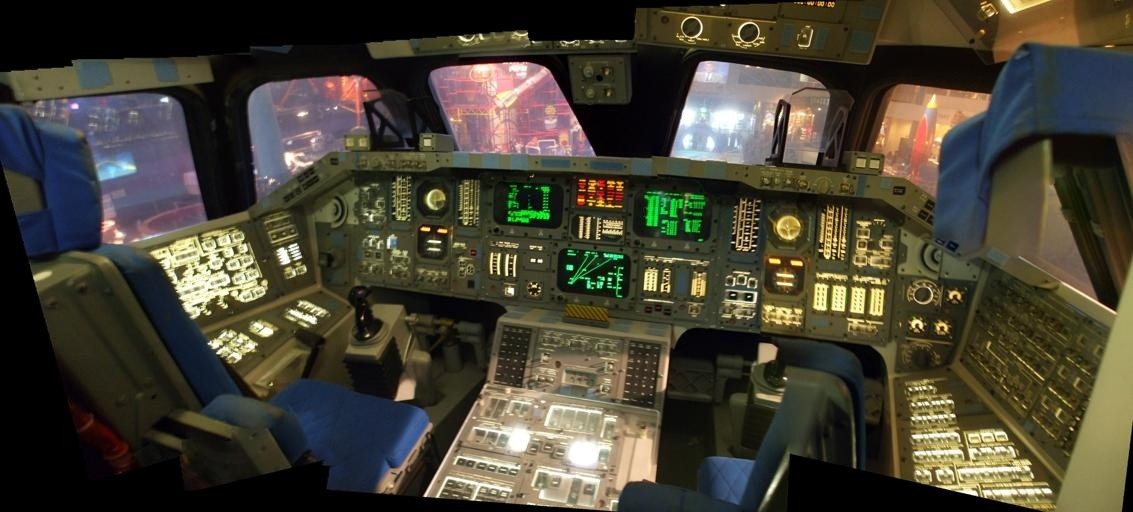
1 100 437 494
618 367 993 512
